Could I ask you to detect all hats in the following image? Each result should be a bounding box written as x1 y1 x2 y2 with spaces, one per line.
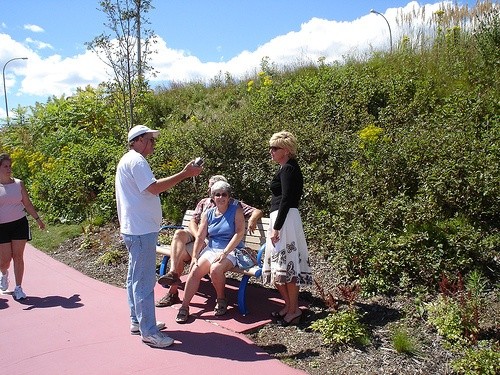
127 125 160 147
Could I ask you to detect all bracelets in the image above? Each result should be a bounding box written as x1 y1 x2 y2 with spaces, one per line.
223 250 228 255
36 217 40 220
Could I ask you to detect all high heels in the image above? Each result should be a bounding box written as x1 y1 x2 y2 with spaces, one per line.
280 314 302 327
271 312 287 320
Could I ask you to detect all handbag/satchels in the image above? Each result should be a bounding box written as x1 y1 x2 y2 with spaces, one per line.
236 247 259 268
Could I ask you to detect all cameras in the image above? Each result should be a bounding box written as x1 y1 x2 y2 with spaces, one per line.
192 157 204 167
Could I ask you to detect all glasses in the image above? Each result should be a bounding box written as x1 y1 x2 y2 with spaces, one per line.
143 138 154 143
216 192 227 197
269 146 283 152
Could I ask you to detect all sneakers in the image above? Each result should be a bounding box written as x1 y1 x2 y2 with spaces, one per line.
130 320 165 335
141 330 174 349
0 270 9 291
14 286 26 300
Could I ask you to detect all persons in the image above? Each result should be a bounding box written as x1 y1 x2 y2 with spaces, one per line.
155 175 263 307
175 181 245 323
115 125 202 346
0 154 44 300
248 132 312 327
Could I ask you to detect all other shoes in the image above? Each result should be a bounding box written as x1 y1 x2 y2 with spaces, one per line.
214 297 230 319
158 270 182 286
175 305 189 324
155 291 180 307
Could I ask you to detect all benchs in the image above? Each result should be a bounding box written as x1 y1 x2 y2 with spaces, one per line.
156 210 270 317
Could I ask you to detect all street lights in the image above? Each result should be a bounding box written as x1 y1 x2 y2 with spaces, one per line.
2 57 28 127
371 9 392 53
